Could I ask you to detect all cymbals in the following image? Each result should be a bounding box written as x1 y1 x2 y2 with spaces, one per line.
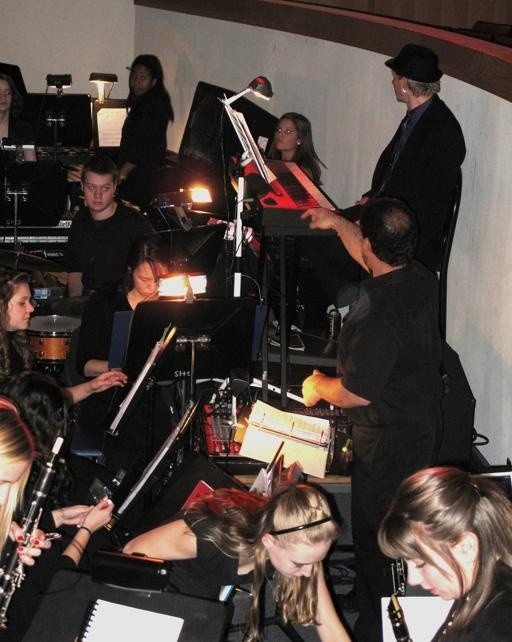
0 248 64 271
51 296 86 318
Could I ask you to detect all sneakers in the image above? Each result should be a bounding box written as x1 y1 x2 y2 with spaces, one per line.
335 590 358 614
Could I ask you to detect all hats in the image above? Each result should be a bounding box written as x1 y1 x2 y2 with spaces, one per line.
383 42 444 84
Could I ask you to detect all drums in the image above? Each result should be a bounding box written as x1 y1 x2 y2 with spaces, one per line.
26 315 82 360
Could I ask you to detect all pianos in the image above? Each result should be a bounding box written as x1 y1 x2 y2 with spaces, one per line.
231 157 341 238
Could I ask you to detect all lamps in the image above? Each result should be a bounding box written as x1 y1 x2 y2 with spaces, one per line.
45 73 73 98
88 70 122 102
222 74 274 296
